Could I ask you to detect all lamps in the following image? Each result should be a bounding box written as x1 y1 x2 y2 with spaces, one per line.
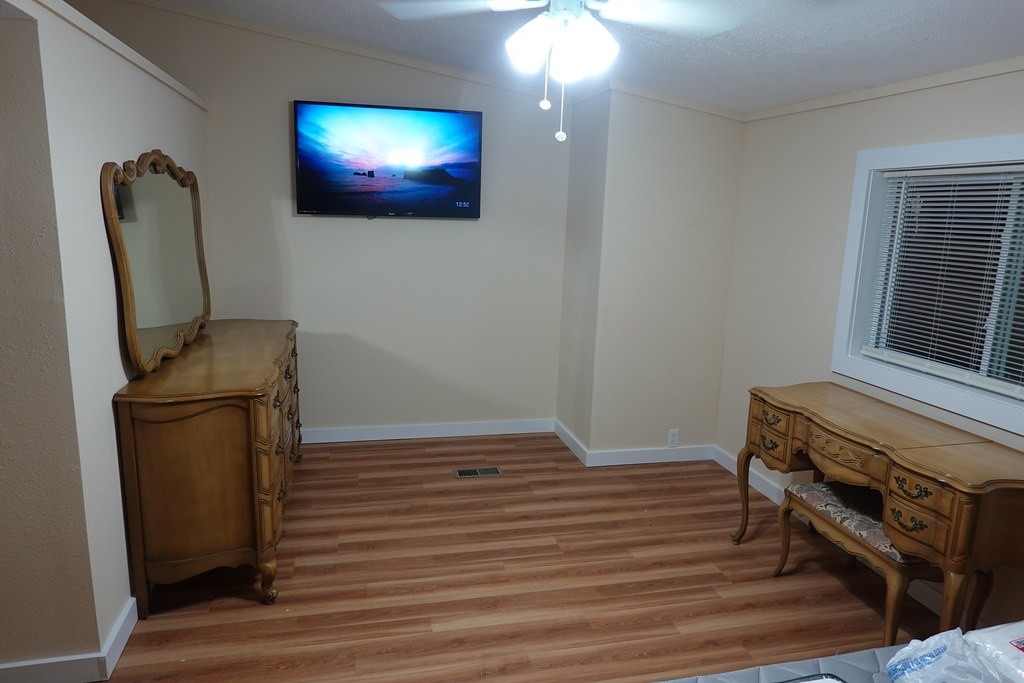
504 0 619 142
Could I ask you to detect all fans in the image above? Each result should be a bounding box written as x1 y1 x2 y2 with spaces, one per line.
378 1 750 40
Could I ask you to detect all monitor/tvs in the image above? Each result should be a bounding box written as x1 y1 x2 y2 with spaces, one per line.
293 99 482 220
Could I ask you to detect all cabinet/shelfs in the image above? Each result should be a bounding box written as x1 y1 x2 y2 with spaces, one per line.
731 379 1024 634
113 319 304 621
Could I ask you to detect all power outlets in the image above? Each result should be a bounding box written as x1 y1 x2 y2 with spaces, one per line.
668 429 679 447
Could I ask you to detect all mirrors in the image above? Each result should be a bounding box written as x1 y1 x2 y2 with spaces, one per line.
100 149 211 375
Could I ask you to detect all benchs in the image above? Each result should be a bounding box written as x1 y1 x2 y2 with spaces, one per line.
770 481 993 649
